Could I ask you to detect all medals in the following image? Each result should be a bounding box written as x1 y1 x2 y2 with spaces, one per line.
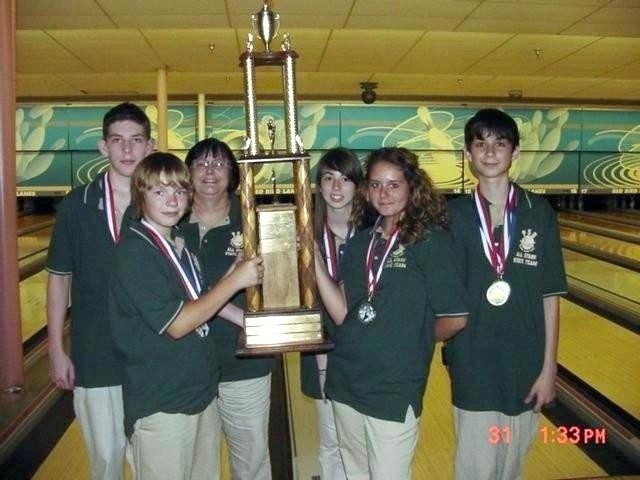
356 301 378 326
484 279 512 307
194 322 210 342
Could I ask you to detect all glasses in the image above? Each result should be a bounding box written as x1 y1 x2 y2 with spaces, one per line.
190 158 233 170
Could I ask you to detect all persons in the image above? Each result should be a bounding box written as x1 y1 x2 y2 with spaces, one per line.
442 107 570 480
298 145 377 480
43 102 158 480
315 145 470 480
108 151 265 479
179 137 275 480
